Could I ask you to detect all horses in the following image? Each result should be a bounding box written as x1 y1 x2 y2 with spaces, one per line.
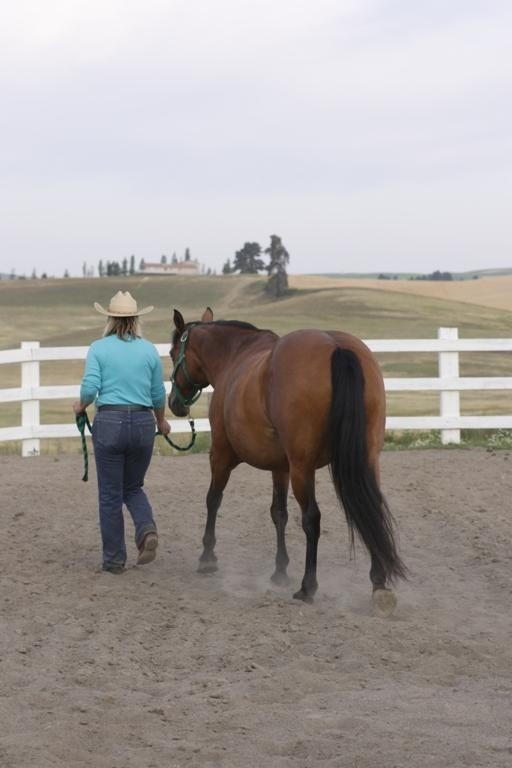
164 306 413 614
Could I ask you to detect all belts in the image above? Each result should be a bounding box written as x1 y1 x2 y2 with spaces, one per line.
98 404 152 410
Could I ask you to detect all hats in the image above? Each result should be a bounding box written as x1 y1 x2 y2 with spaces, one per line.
94 290 153 317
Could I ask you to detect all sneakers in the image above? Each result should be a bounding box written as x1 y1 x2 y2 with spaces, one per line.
137 532 158 564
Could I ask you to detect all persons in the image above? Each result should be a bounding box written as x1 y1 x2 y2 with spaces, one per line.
72 290 172 577
